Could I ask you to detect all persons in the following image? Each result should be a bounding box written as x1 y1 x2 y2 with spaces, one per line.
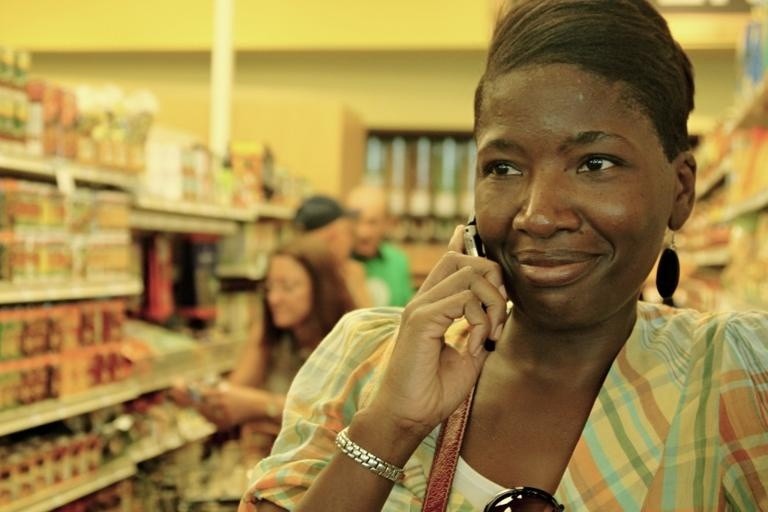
165 235 359 468
236 0 768 511
293 196 374 312
343 185 416 309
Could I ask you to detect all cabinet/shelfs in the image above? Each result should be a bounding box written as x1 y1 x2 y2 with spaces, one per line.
642 70 767 314
5 154 298 510
678 83 764 312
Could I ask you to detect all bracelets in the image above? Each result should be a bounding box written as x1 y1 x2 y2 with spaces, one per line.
335 425 404 485
264 390 279 423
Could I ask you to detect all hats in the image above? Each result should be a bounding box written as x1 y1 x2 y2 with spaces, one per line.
292 195 364 233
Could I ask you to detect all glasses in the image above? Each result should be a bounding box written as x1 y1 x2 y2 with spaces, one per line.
480 485 568 511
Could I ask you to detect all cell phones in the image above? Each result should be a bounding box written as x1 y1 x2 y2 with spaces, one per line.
463 212 496 353
